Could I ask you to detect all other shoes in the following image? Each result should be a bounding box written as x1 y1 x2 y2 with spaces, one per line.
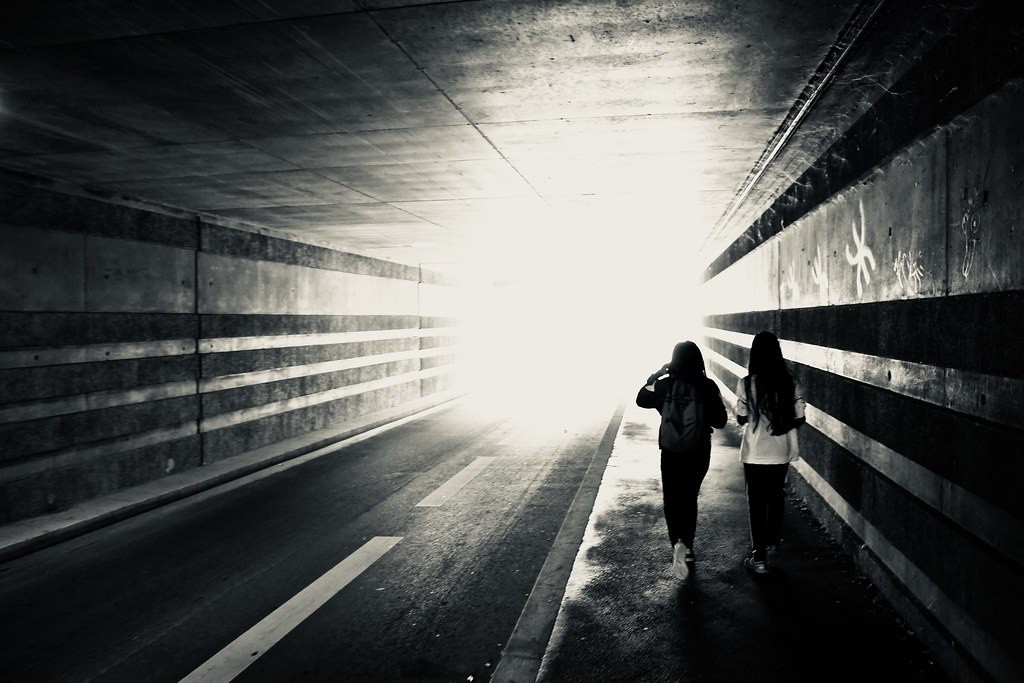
672 543 689 581
685 548 694 562
744 556 767 573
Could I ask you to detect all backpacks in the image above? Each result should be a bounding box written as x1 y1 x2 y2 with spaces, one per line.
658 376 705 454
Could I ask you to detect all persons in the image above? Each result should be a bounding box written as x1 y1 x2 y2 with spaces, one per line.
635 341 727 582
735 332 807 572
